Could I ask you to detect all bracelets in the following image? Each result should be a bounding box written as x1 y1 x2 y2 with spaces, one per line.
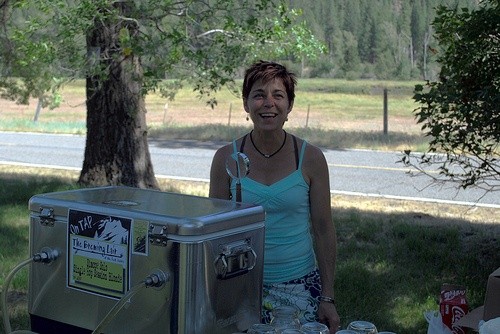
320 296 336 305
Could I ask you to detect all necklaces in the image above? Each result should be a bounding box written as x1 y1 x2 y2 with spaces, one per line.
250 128 287 158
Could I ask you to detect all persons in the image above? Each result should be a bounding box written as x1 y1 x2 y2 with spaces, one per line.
206 60 343 334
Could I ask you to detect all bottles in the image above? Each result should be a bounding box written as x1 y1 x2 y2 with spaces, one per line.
270 306 301 332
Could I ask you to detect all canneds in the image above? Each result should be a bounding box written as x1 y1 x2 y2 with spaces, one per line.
439 282 467 330
245 321 395 334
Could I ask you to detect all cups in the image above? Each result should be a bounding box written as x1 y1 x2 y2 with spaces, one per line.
438 284 468 334
247 321 380 334
